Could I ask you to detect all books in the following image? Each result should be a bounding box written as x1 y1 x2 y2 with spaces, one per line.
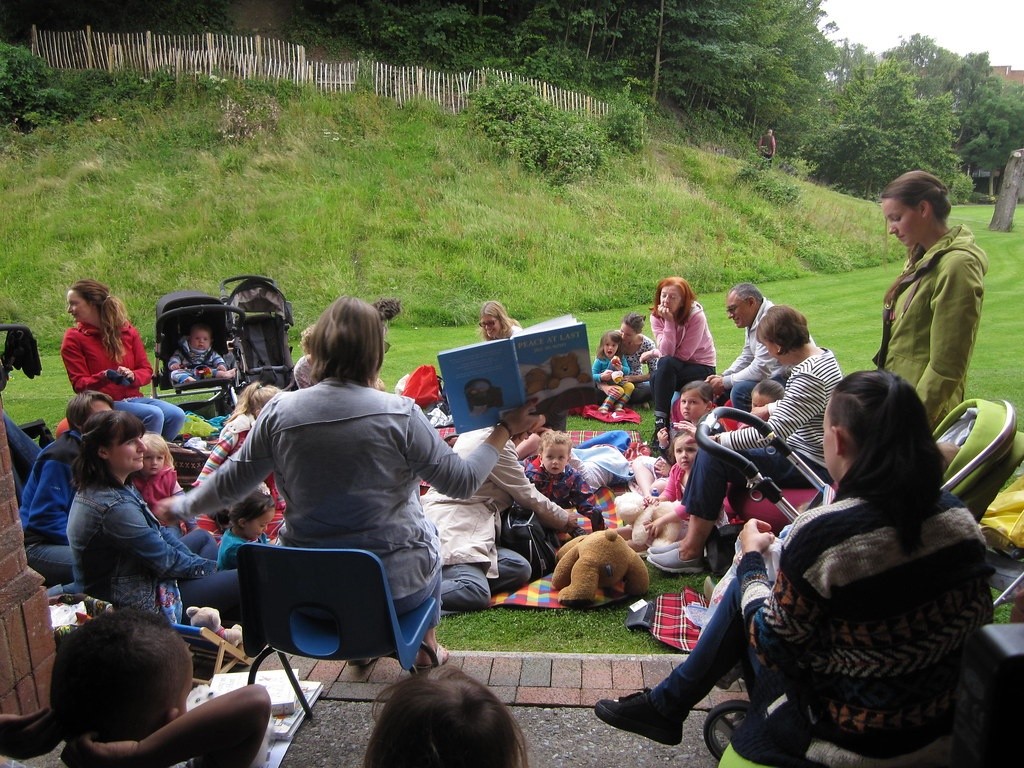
436 314 599 433
209 669 302 715
264 678 325 741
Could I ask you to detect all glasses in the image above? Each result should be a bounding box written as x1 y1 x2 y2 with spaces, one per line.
726 299 748 313
526 431 532 436
480 318 499 326
383 340 391 353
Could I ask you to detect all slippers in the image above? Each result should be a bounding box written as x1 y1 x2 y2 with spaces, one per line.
348 656 379 667
411 644 449 671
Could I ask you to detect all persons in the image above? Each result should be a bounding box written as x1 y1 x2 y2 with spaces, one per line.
3 411 43 503
16 276 995 736
361 666 530 768
758 129 776 169
588 362 996 749
50 609 271 768
870 171 993 442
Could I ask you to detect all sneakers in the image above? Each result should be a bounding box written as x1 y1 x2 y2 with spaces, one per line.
597 688 683 746
647 542 678 555
646 549 704 574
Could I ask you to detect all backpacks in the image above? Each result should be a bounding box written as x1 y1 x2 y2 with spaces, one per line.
501 506 557 583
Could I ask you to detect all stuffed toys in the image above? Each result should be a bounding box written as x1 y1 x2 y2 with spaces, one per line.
182 680 274 767
612 488 689 552
552 529 648 606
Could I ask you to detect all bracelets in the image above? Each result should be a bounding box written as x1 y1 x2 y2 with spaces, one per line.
495 419 514 439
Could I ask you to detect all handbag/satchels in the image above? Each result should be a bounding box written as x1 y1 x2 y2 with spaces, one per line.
169 440 212 486
395 375 409 398
401 365 443 409
626 588 711 652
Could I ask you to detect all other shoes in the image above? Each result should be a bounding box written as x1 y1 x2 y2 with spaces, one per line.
653 418 668 445
704 576 715 600
615 408 624 412
601 408 608 414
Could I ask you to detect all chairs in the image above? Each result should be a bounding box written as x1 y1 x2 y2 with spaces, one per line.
236 544 439 719
170 623 252 687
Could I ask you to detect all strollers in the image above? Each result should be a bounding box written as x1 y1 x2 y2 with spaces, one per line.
694 395 1024 540
149 291 247 418
217 275 298 407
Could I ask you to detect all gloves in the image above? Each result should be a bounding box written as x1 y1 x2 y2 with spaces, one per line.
107 370 131 387
